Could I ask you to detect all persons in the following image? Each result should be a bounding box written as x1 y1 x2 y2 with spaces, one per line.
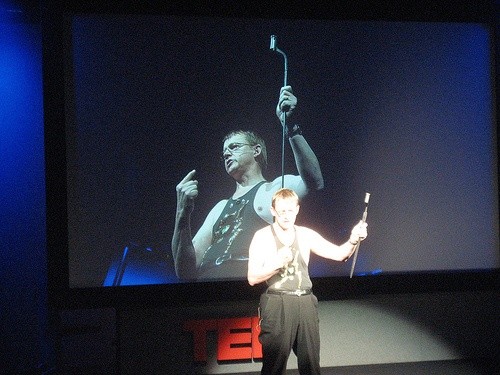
248 189 368 375
171 85 324 282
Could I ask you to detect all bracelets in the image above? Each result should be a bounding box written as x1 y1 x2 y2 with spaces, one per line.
348 239 359 245
284 124 302 138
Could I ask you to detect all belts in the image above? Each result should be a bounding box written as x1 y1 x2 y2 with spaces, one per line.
267 289 311 296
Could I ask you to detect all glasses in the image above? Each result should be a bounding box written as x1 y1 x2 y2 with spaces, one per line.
274 209 297 217
220 142 254 160
